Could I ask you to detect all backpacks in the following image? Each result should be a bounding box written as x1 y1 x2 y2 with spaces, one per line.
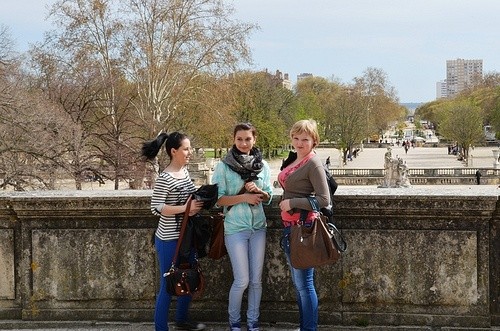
208 213 226 259
163 261 206 298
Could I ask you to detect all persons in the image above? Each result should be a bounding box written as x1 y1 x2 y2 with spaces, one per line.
475 170 481 184
142 132 204 331
448 144 458 155
211 124 271 330
398 139 414 154
278 120 331 331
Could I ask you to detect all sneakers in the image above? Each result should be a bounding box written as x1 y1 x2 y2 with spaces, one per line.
175 321 205 331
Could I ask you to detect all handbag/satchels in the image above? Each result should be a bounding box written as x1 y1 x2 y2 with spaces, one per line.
290 196 348 270
